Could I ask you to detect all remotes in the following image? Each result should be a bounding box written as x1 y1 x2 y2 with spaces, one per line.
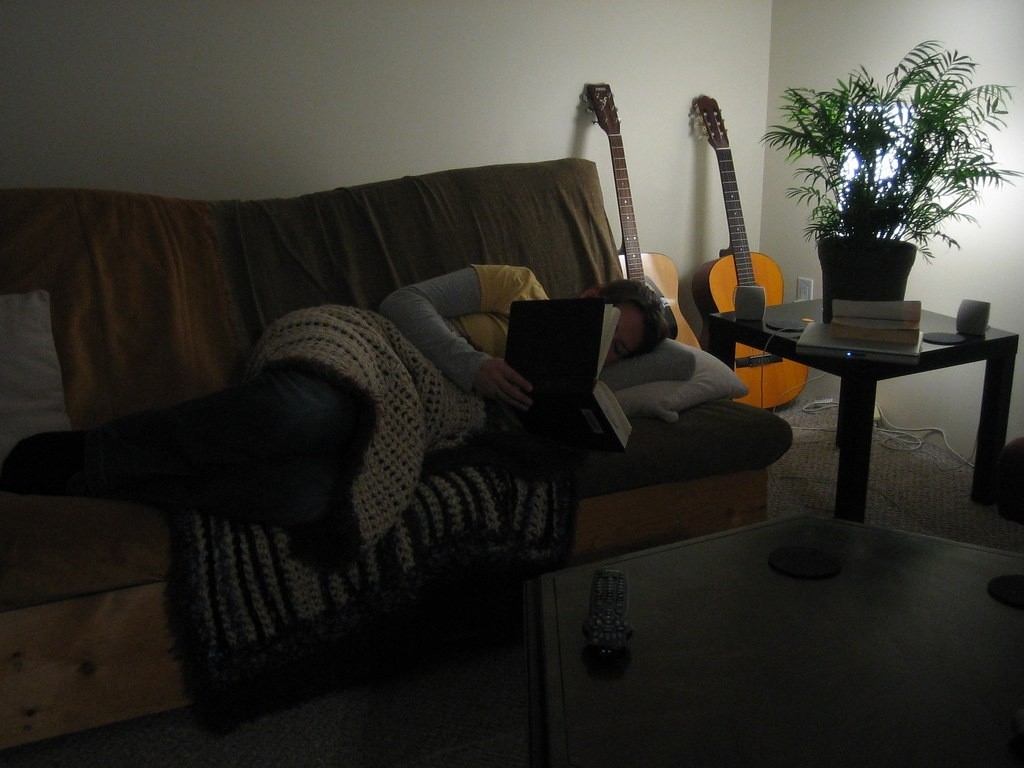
585 569 631 649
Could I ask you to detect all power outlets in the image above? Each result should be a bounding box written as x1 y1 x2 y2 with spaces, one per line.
797 277 814 301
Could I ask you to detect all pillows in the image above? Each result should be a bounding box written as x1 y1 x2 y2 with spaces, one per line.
0 289 73 466
613 338 750 423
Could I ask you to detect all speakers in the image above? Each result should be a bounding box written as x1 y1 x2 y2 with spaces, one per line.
734 286 766 321
955 298 992 335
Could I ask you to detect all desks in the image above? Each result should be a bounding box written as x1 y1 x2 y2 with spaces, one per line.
708 300 1019 522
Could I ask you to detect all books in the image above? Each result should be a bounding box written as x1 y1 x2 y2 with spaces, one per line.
829 294 925 349
503 297 633 457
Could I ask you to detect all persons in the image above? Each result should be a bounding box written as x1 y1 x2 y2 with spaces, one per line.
7 263 672 535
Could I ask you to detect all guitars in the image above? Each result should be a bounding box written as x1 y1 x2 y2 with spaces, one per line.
689 93 809 411
581 83 703 350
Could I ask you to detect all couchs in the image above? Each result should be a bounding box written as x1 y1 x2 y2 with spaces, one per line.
0 158 791 751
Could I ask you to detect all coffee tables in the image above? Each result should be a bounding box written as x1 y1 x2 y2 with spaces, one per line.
518 514 1024 768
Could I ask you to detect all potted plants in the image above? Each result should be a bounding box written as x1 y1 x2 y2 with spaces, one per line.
760 40 1024 326
